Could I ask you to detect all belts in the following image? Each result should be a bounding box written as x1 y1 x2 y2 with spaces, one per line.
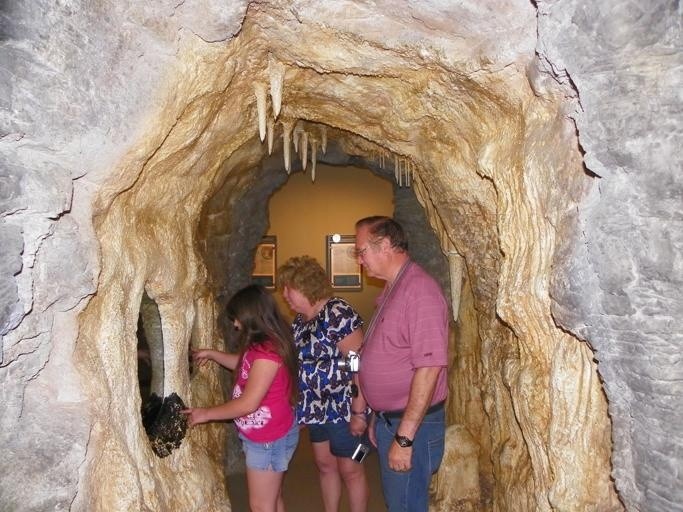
375 398 448 420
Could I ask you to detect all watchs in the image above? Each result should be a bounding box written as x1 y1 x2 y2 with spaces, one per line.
394 433 413 448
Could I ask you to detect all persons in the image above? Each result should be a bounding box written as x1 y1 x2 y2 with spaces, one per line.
137 348 152 368
179 282 300 512
275 254 377 511
352 215 450 511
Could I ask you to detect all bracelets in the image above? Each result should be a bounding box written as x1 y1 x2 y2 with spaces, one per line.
352 409 368 415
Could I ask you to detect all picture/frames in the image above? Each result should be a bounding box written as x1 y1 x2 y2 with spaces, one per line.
252 235 278 292
326 234 363 293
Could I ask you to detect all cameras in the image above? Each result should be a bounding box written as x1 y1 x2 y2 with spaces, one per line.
351 444 370 464
